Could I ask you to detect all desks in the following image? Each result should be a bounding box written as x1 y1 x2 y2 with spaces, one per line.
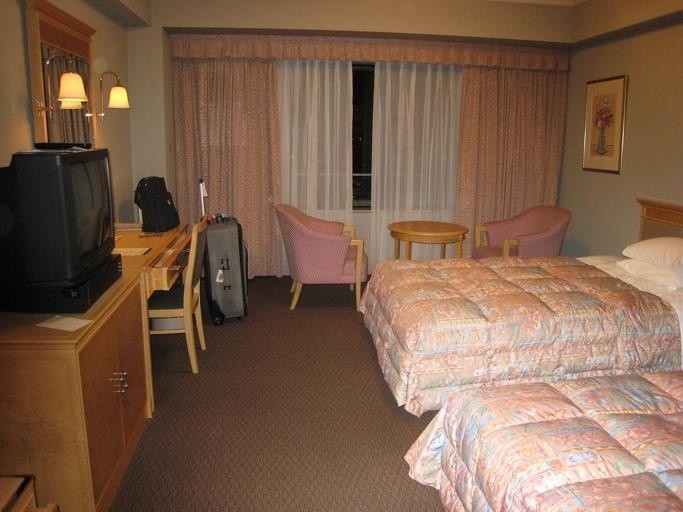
0 474 36 511
1 223 189 511
388 221 469 260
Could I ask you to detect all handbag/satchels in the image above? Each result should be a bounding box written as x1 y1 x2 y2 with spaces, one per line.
134 176 179 233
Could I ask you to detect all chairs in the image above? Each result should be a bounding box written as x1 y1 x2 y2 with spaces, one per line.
146 218 209 374
471 205 571 258
273 205 368 311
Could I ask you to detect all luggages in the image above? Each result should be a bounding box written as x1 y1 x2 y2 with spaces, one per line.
199 179 248 325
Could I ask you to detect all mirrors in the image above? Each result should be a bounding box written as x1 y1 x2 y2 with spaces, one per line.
23 0 96 142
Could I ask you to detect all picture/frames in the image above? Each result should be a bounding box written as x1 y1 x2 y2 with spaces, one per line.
581 74 629 173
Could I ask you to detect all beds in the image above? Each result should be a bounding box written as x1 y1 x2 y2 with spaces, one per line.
403 370 683 511
359 195 683 416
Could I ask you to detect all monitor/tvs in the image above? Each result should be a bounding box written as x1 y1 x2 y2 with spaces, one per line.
0 147 122 314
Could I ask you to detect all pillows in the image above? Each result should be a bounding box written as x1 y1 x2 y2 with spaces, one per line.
616 258 683 288
621 237 682 275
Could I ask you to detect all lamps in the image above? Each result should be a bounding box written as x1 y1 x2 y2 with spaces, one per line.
36 51 89 114
98 70 131 117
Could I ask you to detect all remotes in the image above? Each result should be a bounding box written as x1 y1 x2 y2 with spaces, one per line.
34 142 92 149
